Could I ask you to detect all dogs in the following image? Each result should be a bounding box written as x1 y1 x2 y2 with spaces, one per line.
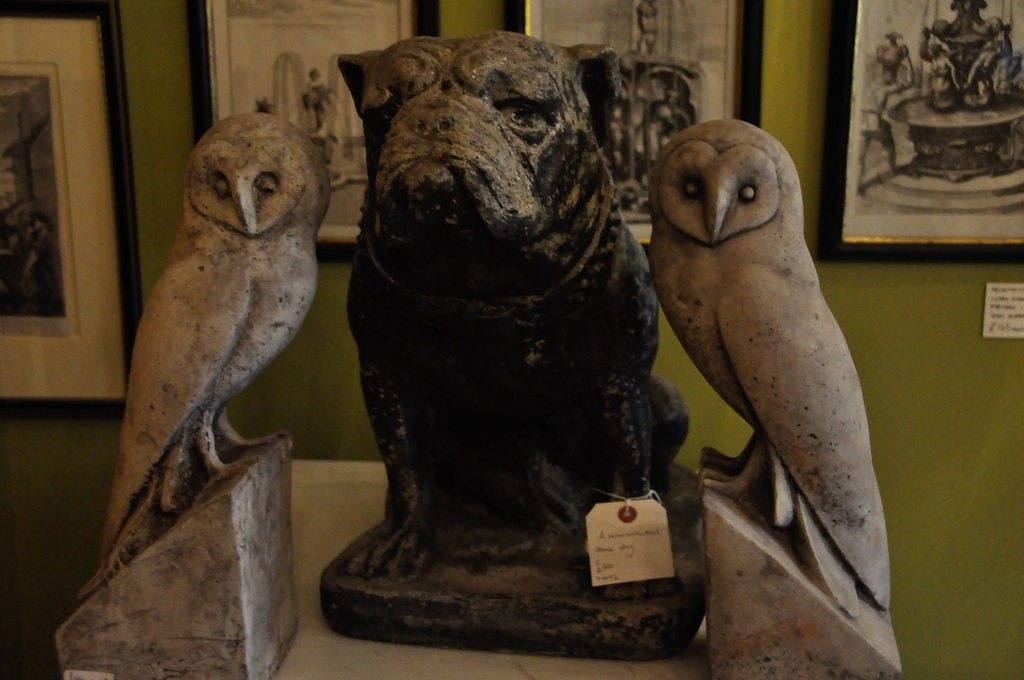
338 31 703 596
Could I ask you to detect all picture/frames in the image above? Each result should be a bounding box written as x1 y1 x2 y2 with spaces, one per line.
504 0 764 255
0 0 143 422
818 0 1024 264
185 0 440 262
